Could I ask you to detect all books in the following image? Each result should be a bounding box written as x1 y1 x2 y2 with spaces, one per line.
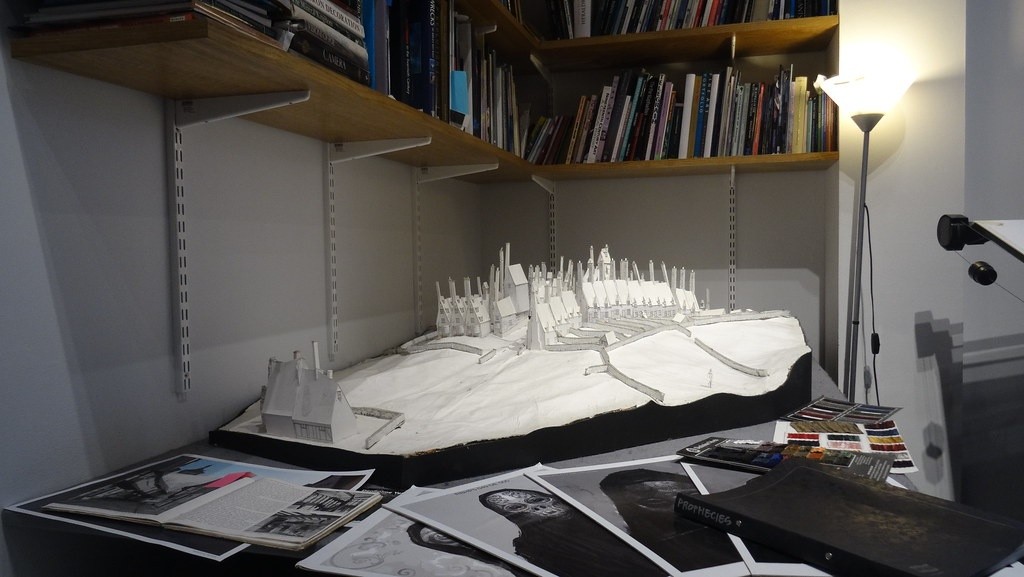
675 460 1024 577
675 436 908 488
5 0 839 164
43 473 383 552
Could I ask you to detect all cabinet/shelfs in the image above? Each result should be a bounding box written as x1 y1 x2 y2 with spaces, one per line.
1 0 842 189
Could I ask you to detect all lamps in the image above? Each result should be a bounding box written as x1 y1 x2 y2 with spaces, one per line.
820 72 918 405
